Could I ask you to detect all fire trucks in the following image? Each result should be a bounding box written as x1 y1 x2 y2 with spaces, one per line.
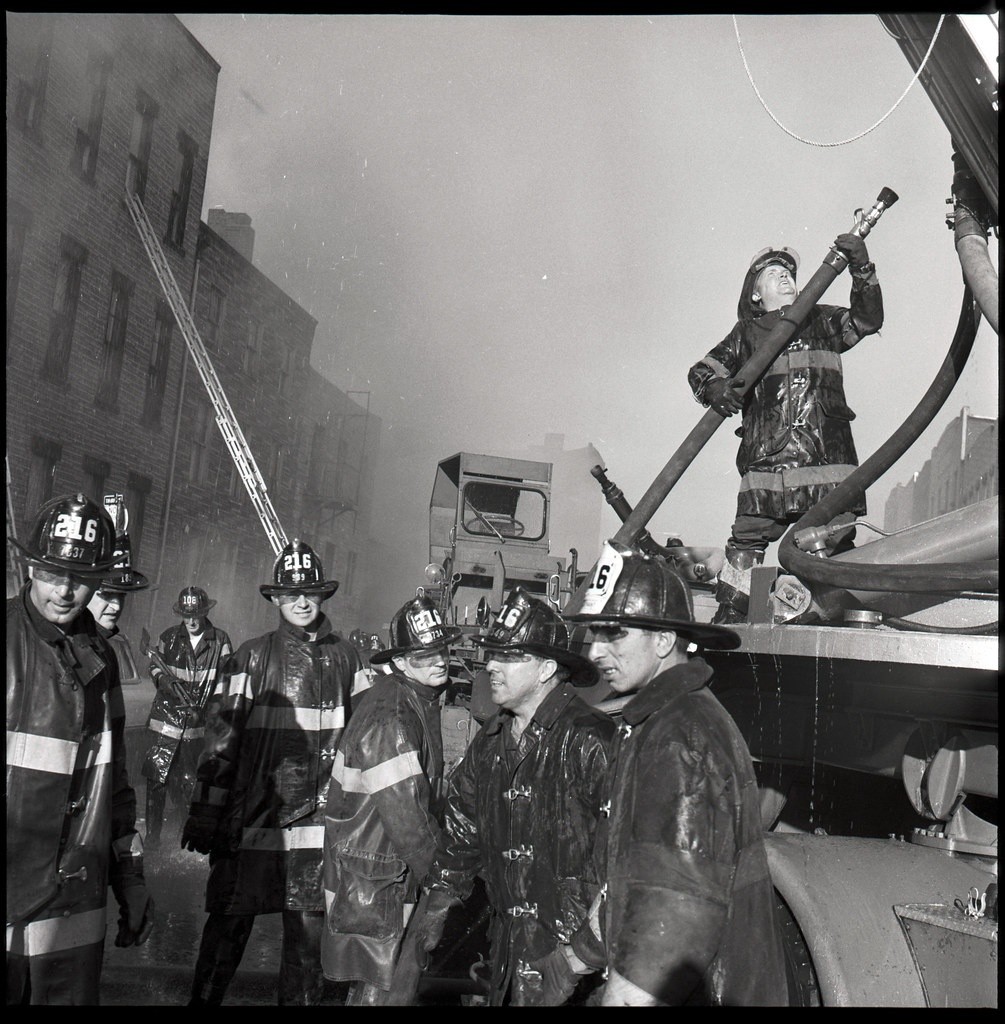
417 15 997 1008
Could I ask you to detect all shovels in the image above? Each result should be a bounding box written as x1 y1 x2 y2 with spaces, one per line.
136 625 201 722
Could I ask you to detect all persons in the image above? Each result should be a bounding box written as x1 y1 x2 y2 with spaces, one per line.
6 493 155 1007
86 528 149 640
688 234 885 625
321 596 463 1006
415 587 616 1006
522 555 788 1005
182 541 365 1006
332 629 386 670
141 586 234 851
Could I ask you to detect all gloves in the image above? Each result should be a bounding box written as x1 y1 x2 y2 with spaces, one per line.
181 815 218 855
508 942 582 1006
114 886 158 948
413 888 466 974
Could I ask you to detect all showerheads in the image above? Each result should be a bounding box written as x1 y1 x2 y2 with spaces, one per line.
590 464 625 505
834 184 899 239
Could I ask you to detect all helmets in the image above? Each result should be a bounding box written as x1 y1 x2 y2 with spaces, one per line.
734 247 798 318
260 538 340 601
7 491 127 572
370 598 463 664
561 541 739 652
171 586 216 619
467 586 601 690
101 533 149 591
